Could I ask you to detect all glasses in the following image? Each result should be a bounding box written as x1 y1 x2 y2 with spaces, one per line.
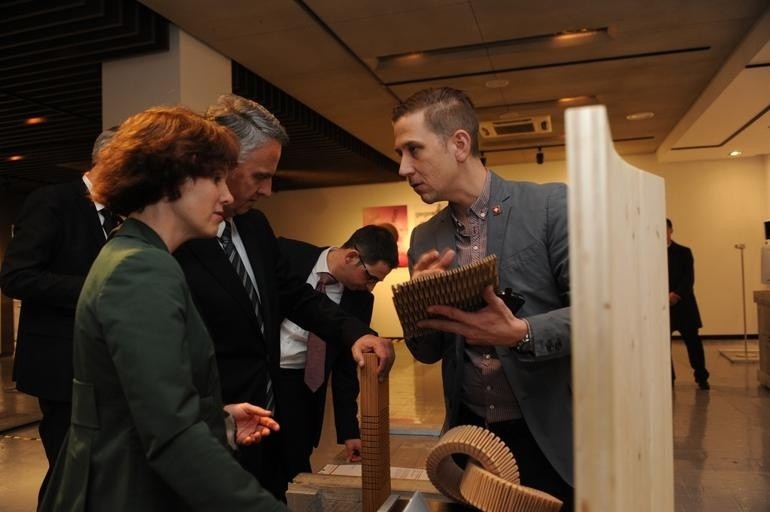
354 244 379 285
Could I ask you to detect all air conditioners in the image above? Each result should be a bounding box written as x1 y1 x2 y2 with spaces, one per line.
473 115 552 138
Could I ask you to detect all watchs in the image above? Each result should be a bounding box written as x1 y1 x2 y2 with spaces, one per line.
513 331 532 355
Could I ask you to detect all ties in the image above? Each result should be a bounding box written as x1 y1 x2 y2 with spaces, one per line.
219 222 278 419
100 208 123 236
304 272 339 392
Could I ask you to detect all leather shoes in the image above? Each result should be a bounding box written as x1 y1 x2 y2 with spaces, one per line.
695 379 710 390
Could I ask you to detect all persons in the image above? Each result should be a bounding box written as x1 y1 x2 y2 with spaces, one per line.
13 130 126 512
392 86 572 511
272 226 400 477
37 109 286 512
665 219 712 391
170 95 396 512
